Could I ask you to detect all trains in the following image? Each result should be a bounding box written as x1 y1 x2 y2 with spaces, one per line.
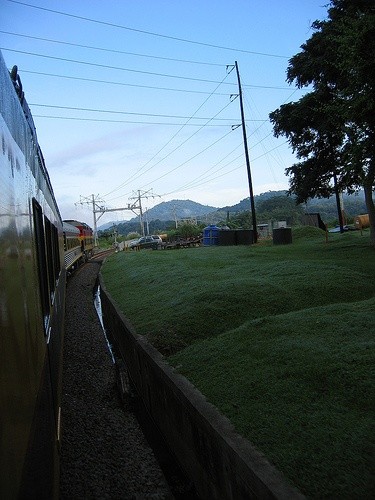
0 49 95 500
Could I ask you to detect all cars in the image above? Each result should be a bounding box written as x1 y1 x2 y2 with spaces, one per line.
130 235 163 249
